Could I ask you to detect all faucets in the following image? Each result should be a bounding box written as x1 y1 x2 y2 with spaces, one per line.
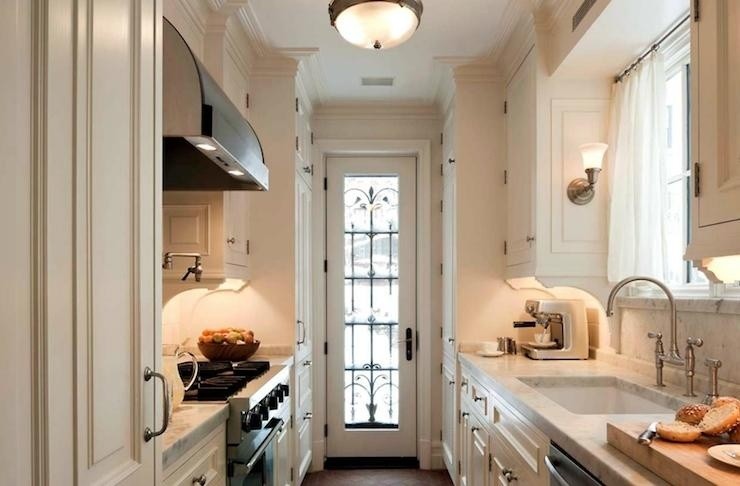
606 276 678 354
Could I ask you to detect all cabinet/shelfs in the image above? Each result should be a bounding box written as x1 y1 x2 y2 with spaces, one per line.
273 369 294 486
441 353 466 486
441 90 502 177
227 172 310 353
162 34 249 280
0 0 170 486
441 168 501 346
247 76 314 192
681 0 740 261
164 420 226 486
458 361 550 486
290 350 313 483
503 46 612 280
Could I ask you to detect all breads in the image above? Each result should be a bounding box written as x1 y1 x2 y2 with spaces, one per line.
655 396 740 443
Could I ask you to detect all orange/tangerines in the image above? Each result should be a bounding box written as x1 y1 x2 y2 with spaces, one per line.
198 328 254 343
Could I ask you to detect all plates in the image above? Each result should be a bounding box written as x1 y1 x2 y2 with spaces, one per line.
476 351 504 357
708 444 740 467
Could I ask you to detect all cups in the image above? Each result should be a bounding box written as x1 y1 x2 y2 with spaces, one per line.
482 341 499 352
497 336 517 355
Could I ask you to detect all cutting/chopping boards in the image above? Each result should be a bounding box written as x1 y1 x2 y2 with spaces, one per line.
606 421 740 486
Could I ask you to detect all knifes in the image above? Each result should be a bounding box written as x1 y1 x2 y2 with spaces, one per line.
639 421 660 445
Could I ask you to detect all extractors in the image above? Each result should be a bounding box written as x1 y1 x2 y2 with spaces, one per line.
162 13 269 192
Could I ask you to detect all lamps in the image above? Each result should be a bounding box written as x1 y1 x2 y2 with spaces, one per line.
567 142 608 206
328 0 423 50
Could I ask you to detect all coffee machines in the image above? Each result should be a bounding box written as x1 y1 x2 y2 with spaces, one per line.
512 298 590 360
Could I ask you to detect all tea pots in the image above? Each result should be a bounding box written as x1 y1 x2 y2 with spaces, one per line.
160 351 198 411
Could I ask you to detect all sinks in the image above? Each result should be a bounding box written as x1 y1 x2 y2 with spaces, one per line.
515 375 688 417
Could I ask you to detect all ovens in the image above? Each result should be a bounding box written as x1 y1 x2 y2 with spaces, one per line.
226 398 284 486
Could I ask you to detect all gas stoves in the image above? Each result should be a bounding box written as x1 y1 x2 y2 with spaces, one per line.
167 362 291 444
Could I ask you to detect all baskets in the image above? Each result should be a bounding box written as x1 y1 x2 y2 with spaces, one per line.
197 340 260 361
